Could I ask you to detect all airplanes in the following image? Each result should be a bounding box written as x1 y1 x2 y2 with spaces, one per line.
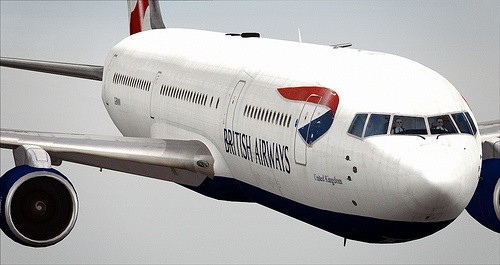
1 1 500 247
